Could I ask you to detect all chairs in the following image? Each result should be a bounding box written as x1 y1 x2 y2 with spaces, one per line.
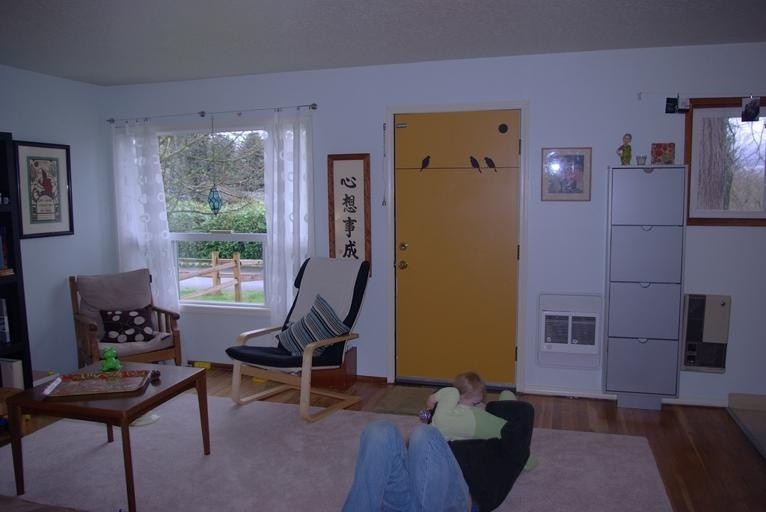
224 257 375 424
67 269 186 370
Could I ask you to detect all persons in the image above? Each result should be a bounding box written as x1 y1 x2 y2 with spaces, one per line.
396 386 538 472
615 131 633 165
31 166 53 200
341 386 535 512
544 154 581 191
426 371 488 426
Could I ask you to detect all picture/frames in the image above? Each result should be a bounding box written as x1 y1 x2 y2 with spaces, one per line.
13 140 78 241
682 94 766 228
327 151 373 277
539 144 591 201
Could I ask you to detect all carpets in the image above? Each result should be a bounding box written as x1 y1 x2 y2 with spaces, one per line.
1 394 677 511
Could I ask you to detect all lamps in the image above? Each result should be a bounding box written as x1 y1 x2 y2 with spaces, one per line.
207 115 223 217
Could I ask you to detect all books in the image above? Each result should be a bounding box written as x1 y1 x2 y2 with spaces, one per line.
0 237 5 270
0 297 10 344
0 224 11 270
0 268 14 277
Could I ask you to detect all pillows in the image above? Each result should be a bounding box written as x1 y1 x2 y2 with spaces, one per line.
97 302 159 344
276 294 351 361
